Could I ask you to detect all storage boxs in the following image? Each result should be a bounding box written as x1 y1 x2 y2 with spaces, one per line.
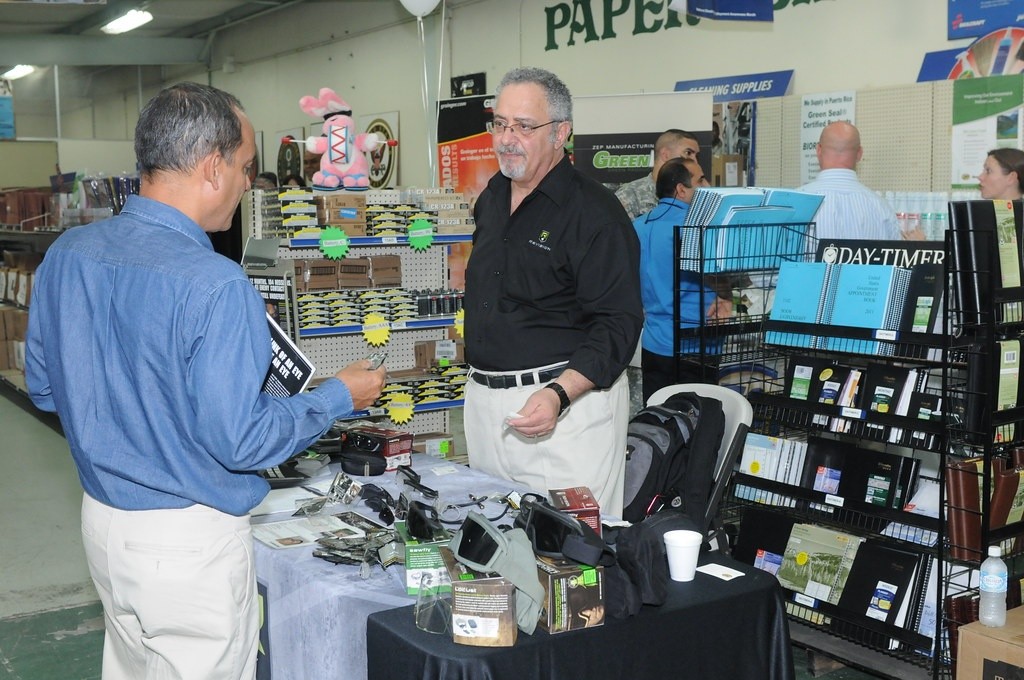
0 186 63 373
452 581 519 648
260 188 467 468
532 559 604 635
547 484 603 540
956 606 1024 680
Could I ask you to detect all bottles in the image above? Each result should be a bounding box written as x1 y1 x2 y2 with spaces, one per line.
978 547 1008 627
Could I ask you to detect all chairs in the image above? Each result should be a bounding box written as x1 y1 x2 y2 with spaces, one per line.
644 383 752 558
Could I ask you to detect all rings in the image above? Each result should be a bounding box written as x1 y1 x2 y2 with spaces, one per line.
534 434 537 439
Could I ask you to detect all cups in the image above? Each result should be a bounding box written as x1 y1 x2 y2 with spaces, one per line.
664 530 702 582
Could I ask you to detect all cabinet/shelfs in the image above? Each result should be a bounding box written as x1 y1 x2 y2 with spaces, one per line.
669 221 819 394
696 255 987 680
935 191 1024 680
1 224 65 439
279 232 467 419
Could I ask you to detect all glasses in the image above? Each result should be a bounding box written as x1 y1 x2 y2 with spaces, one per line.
448 511 503 572
365 497 394 526
407 501 443 538
486 120 563 136
514 493 584 558
385 465 439 498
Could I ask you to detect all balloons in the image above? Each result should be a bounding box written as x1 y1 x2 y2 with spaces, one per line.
401 0 441 18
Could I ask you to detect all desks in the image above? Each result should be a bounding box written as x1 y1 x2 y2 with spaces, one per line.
248 452 545 680
364 560 794 680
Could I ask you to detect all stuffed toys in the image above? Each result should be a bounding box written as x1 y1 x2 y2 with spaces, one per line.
299 87 378 188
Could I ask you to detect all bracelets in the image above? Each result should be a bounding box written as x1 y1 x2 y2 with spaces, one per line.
545 383 571 418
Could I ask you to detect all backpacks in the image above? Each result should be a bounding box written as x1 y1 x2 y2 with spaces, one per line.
623 390 724 520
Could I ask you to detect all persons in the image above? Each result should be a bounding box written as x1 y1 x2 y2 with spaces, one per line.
23 82 388 680
613 127 735 408
792 120 904 240
462 67 644 521
253 150 322 189
899 148 1024 241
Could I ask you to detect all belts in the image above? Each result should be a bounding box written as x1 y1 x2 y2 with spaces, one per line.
473 363 569 389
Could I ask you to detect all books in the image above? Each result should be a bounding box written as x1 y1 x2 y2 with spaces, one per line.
947 199 1023 323
680 187 826 274
261 312 316 397
944 446 1024 561
777 356 964 452
777 522 946 659
733 432 947 547
944 589 979 680
764 263 913 358
965 336 1024 443
